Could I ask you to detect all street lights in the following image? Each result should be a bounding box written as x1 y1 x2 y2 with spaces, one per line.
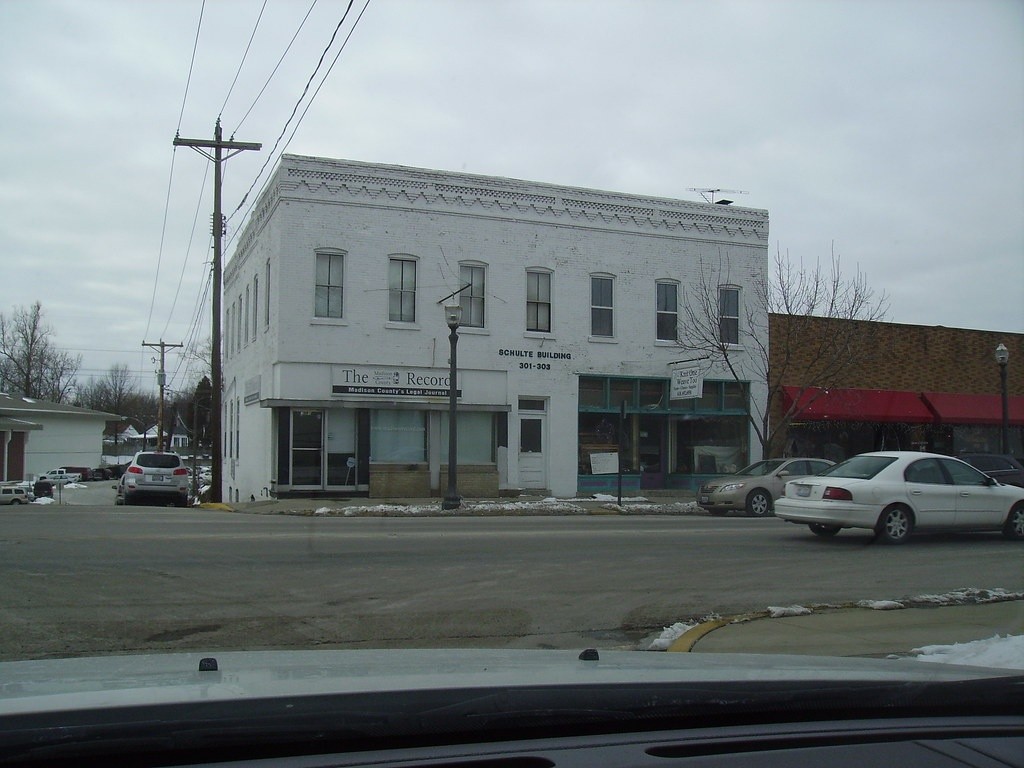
442 299 464 510
996 344 1011 455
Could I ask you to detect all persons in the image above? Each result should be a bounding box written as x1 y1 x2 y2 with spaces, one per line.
34 475 53 500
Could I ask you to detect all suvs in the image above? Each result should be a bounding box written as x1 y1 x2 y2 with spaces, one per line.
123 449 190 506
951 450 1024 487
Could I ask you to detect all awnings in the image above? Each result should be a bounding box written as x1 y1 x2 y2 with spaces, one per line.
779 383 1024 423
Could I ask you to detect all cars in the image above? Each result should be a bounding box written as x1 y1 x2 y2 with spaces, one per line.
773 450 1023 544
696 457 839 513
187 461 214 490
0 463 126 507
